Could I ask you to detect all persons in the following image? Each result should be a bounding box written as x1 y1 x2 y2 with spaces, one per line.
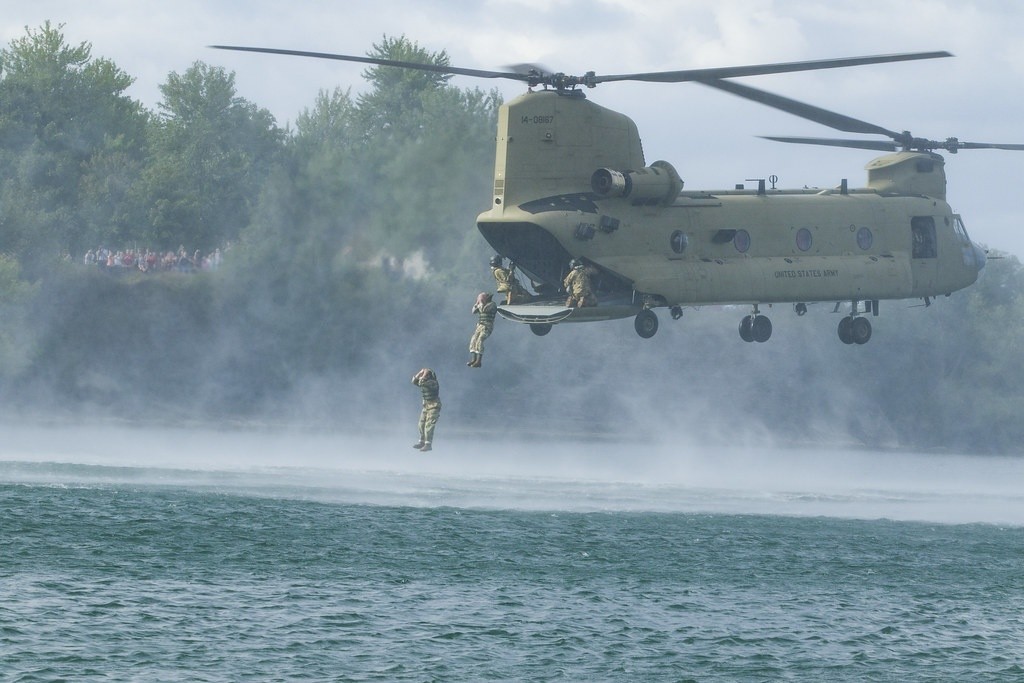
466 292 497 367
489 254 532 305
411 368 442 451
563 258 598 309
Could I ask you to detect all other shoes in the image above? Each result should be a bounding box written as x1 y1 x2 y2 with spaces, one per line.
421 442 431 450
566 296 572 308
413 440 424 447
578 296 585 307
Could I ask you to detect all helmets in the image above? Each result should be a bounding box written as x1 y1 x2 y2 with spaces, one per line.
490 255 502 265
569 259 582 270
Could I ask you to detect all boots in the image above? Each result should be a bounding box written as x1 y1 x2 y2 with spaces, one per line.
465 352 476 365
471 354 482 368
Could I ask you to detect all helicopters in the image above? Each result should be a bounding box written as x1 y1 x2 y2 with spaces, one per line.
204 45 1024 345
